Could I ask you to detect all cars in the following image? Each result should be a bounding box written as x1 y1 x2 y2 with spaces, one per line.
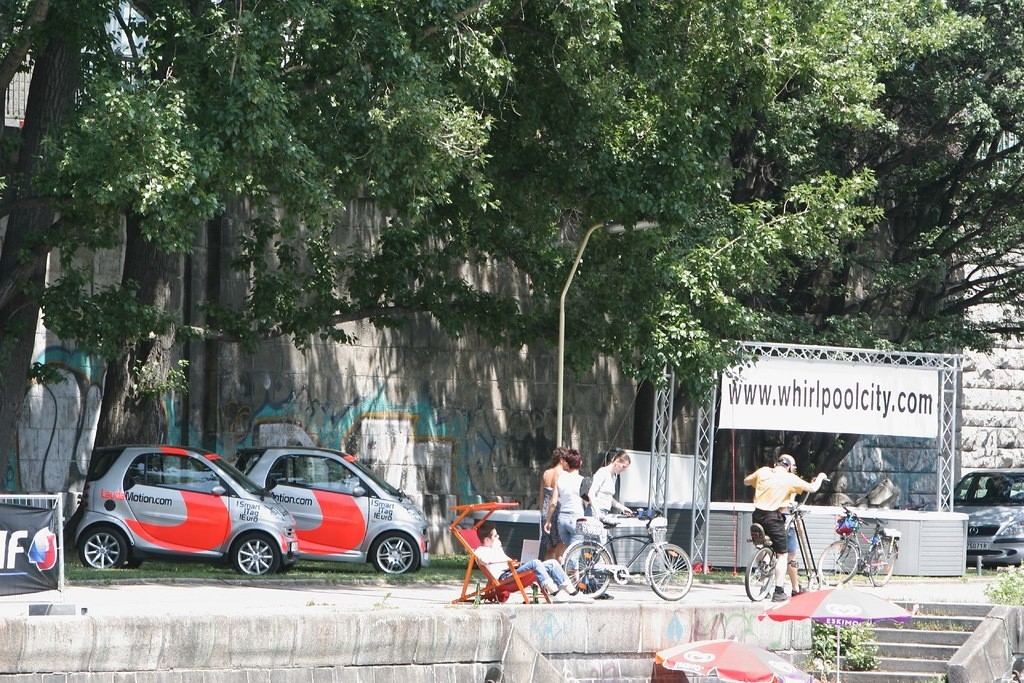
234 447 429 576
942 469 1024 568
74 445 299 576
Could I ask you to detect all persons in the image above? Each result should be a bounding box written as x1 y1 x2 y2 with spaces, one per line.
744 454 827 602
579 448 631 599
542 449 592 587
539 446 567 566
474 523 595 604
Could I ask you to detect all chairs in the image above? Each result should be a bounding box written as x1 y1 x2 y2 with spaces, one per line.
983 477 1015 502
448 502 551 605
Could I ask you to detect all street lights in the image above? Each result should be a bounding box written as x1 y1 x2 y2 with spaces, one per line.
557 220 662 448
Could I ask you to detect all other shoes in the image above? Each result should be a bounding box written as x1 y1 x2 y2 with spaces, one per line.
772 592 790 602
583 584 614 600
792 584 809 597
760 586 770 600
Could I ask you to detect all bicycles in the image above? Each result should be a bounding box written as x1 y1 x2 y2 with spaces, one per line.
818 507 899 587
561 510 696 601
745 477 830 601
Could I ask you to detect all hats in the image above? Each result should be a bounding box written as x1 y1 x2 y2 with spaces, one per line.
774 456 791 473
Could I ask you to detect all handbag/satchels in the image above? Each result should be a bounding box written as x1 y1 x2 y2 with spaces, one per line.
580 476 593 502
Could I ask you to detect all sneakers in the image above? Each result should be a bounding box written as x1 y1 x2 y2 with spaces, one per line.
570 590 594 603
553 589 577 603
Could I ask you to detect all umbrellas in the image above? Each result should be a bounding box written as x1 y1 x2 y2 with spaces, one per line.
759 581 913 683
654 638 814 683
